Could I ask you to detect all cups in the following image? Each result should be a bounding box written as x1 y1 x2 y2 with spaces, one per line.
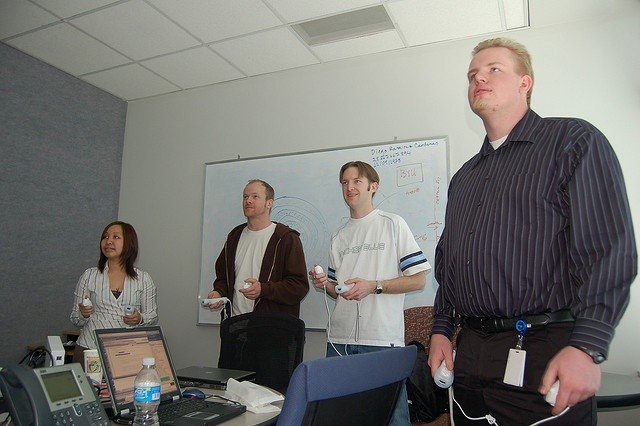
83 348 103 385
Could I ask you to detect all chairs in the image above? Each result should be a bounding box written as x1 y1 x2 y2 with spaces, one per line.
404 305 462 426
275 345 418 425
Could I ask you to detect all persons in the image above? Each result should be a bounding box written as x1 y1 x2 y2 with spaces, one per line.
69 221 158 374
309 160 433 426
427 36 639 426
203 179 310 370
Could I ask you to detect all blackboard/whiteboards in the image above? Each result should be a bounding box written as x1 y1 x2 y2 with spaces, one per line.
197 138 450 333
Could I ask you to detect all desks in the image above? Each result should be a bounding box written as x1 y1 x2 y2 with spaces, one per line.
180 382 285 426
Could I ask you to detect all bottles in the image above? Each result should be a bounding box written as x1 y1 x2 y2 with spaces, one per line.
132 358 162 426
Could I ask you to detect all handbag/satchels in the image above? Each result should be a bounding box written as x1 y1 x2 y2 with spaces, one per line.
405 341 449 424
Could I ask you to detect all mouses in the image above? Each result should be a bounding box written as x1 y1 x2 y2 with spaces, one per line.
182 389 205 399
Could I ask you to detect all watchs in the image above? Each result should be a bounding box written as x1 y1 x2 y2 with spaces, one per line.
374 280 383 294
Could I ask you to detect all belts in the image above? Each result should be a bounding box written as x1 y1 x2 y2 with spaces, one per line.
462 310 576 335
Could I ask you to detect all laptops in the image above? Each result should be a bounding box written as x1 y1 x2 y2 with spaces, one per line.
94 326 246 425
176 366 256 390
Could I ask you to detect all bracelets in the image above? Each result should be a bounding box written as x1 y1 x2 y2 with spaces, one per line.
139 314 142 324
570 345 605 364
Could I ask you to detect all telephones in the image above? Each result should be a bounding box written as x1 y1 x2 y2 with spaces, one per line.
0 363 109 426
